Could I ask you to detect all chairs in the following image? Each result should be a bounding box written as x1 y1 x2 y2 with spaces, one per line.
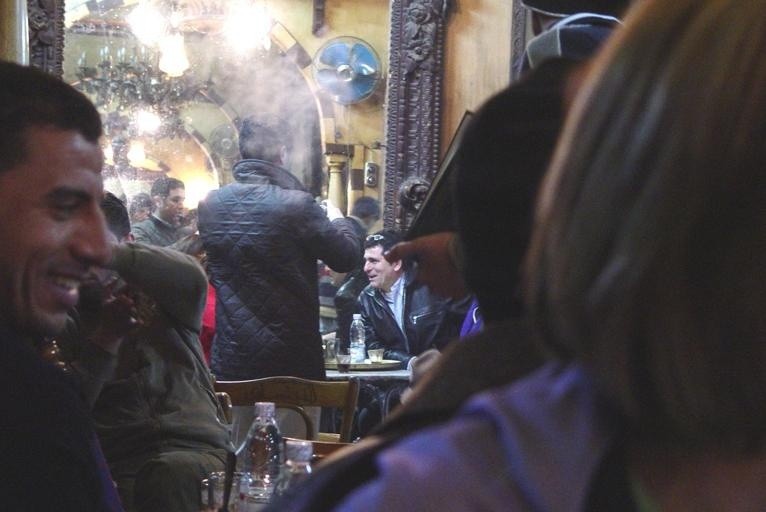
189 334 415 511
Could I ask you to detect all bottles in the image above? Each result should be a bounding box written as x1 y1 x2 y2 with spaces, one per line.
349 313 366 363
202 399 315 512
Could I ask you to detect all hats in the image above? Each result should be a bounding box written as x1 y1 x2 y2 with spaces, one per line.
516 12 625 85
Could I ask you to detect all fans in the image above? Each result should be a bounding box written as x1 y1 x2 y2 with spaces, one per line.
307 34 389 108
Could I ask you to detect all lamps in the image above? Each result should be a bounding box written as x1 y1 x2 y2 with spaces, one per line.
74 43 200 109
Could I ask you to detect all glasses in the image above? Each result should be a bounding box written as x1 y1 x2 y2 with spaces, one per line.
364 234 390 243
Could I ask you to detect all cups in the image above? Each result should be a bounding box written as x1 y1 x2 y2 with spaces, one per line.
336 354 352 375
367 348 385 365
